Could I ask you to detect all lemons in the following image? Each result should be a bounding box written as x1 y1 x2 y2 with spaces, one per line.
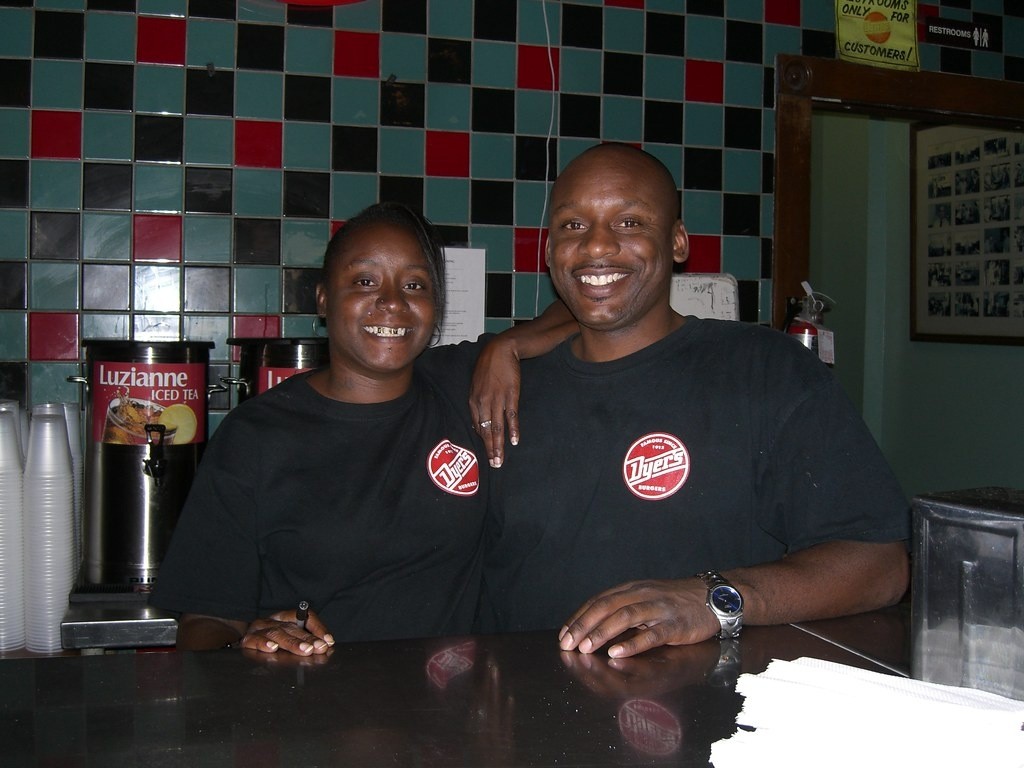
159 403 197 444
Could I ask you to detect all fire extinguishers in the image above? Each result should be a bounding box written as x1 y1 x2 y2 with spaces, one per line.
783 295 832 357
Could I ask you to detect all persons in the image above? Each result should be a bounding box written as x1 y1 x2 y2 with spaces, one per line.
468 610 903 768
482 140 913 659
147 200 583 657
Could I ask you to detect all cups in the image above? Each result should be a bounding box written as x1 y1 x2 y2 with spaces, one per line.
1 397 87 655
101 396 178 445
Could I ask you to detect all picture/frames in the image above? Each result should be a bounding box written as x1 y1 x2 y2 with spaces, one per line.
909 122 1023 345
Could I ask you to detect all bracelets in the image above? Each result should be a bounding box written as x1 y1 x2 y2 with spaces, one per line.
221 640 240 648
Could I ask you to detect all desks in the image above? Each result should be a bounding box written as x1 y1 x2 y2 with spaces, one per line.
1 602 911 768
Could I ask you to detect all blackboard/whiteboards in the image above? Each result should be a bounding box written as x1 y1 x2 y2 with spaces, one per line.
669 273 740 321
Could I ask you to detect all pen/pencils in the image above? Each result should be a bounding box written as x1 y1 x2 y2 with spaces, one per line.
296 601 309 629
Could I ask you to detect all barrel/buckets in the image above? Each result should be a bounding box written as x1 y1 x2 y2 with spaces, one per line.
76 334 217 597
223 336 329 410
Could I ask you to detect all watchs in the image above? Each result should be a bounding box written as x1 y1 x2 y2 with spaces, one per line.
703 638 742 688
695 567 743 639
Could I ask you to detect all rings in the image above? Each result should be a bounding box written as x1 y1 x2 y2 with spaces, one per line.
480 420 491 428
472 424 480 430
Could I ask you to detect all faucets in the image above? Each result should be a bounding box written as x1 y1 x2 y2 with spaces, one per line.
142 424 169 488
